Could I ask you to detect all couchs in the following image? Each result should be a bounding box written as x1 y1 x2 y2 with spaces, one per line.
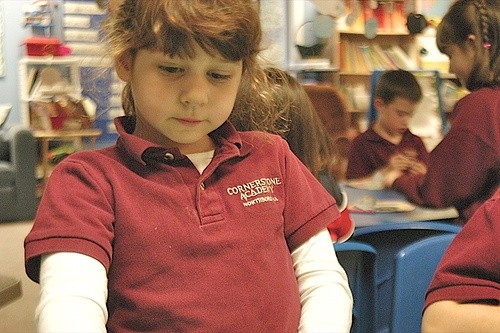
0 126 40 223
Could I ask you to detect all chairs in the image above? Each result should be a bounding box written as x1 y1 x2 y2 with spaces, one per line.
334 222 464 333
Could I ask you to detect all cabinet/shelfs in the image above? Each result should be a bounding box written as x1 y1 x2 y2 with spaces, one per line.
337 29 457 136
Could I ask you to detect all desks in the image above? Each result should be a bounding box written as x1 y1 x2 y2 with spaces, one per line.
341 180 459 227
33 130 104 201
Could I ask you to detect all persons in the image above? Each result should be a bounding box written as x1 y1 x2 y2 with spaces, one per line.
382 0 500 228
344 69 430 180
228 66 354 245
24 0 354 333
420 186 500 333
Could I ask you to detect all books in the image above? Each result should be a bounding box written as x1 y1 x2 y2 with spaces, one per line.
339 38 421 72
361 0 410 34
341 82 372 133
